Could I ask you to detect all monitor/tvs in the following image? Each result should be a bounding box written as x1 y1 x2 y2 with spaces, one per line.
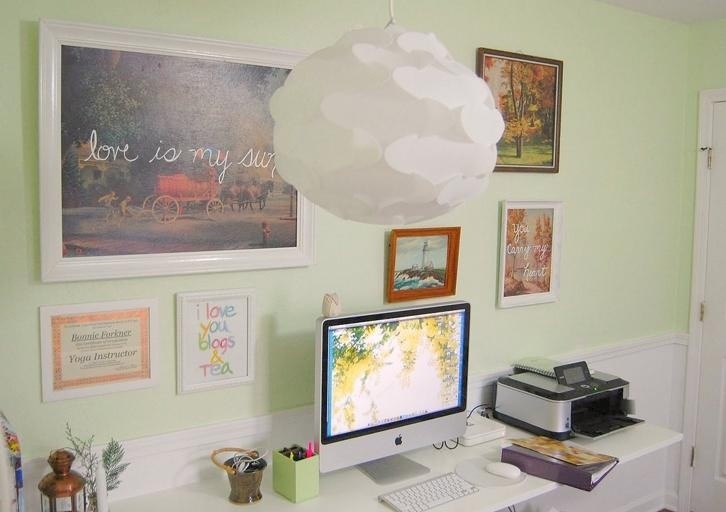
314 299 470 487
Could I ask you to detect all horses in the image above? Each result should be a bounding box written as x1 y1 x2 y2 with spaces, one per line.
240 179 274 210
221 179 256 210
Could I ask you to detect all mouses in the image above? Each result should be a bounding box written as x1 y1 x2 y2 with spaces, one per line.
485 462 521 481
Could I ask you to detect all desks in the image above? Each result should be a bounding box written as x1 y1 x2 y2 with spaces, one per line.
104 412 680 512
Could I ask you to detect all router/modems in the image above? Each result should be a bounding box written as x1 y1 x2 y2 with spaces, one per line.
451 410 507 447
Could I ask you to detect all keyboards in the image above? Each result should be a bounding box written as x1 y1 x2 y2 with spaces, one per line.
377 471 479 512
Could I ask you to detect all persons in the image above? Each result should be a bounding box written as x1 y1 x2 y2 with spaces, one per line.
118 192 136 218
98 191 120 223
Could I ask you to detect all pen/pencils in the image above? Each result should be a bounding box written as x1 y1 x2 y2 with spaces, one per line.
277 439 312 461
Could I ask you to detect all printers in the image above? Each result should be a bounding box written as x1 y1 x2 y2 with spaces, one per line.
492 357 646 442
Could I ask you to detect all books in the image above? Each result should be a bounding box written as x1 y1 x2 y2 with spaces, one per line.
573 413 645 441
509 355 595 379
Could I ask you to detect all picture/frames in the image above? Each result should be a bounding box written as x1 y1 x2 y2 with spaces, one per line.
472 46 563 175
39 299 159 404
386 225 461 303
37 15 316 283
175 288 253 396
497 198 563 309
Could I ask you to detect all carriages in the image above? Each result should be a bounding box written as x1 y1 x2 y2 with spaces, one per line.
143 161 273 224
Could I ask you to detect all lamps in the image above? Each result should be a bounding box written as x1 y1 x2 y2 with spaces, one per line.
266 1 505 228
